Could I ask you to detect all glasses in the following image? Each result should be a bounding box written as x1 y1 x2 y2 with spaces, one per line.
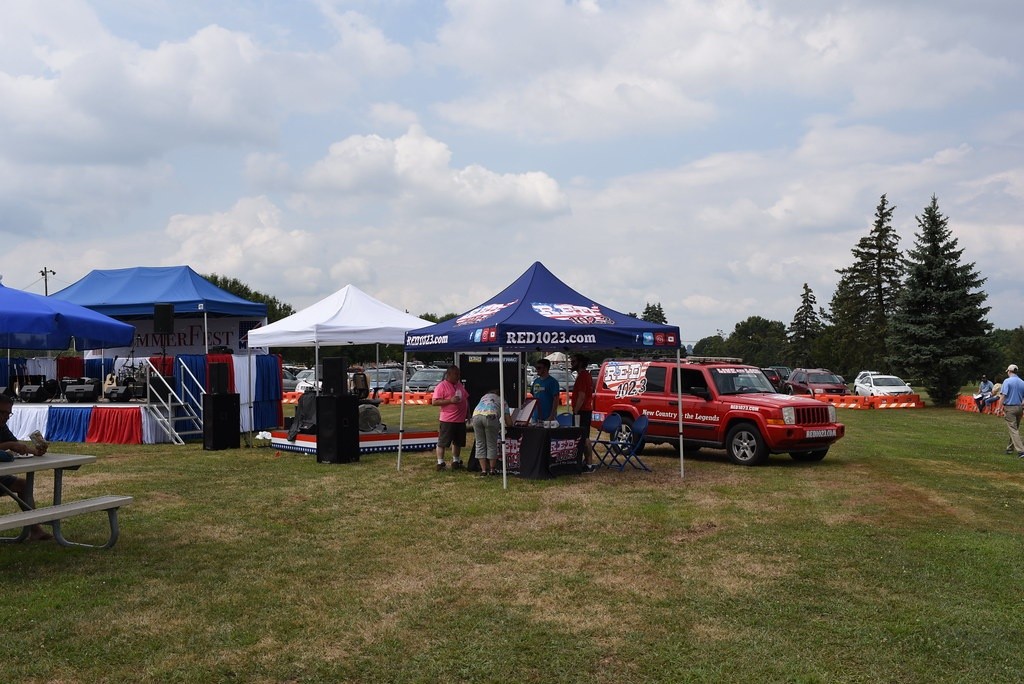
0 410 13 417
536 367 544 370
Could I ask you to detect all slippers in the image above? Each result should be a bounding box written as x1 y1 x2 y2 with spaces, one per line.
30 534 55 540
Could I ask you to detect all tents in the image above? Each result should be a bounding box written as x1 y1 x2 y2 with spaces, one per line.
396 260 684 489
48 265 268 398
247 284 437 447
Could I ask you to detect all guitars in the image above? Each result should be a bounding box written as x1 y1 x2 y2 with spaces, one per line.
103 354 118 393
15 368 31 396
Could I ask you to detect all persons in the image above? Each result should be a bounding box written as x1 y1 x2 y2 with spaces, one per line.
0 393 55 541
530 359 559 421
974 374 1002 415
472 388 513 477
997 364 1024 459
432 365 470 472
571 355 596 474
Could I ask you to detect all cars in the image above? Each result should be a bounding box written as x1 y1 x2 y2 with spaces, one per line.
296 370 314 381
283 370 300 393
549 370 575 392
854 371 879 393
527 366 540 387
405 368 447 393
770 366 793 383
295 372 322 393
854 376 914 409
836 375 847 386
366 370 403 393
761 367 784 394
572 371 578 379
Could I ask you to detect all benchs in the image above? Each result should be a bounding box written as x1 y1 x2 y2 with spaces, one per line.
0 494 134 550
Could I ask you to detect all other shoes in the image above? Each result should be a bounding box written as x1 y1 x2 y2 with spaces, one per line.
489 470 497 475
1002 449 1014 455
480 471 487 477
1017 451 1024 459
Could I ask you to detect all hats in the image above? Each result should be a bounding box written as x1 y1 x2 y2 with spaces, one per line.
1005 364 1018 372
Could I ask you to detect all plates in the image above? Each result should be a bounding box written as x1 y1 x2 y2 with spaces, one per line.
14 453 34 459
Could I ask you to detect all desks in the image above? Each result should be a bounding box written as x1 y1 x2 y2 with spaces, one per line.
469 427 585 475
0 453 118 552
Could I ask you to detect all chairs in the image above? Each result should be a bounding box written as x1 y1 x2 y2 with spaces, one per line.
591 412 625 468
603 415 649 472
555 412 575 426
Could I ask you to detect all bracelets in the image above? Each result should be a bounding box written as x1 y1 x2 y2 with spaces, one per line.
997 408 1001 414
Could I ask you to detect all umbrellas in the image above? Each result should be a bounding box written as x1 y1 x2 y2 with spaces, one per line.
0 275 135 351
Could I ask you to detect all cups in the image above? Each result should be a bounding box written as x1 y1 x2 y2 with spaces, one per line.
544 421 550 428
30 430 45 448
575 415 580 427
551 421 559 428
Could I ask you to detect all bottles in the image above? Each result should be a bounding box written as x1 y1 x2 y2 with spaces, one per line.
455 389 462 402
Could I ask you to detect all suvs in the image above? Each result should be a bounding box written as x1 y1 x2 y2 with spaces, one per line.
591 356 846 467
785 368 851 399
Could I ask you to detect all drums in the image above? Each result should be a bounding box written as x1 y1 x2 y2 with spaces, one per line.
119 371 132 381
122 377 141 397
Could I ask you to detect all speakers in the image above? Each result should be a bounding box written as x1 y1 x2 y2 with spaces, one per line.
65 385 98 402
202 363 241 450
106 382 147 402
315 357 360 464
20 385 48 403
154 303 174 334
150 376 176 402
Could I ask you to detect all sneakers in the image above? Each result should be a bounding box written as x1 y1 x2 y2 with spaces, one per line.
436 462 449 472
581 465 595 473
451 460 467 472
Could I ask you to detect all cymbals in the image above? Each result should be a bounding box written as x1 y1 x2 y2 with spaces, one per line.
123 365 140 370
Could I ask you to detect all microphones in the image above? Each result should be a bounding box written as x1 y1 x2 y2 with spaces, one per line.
136 337 141 339
